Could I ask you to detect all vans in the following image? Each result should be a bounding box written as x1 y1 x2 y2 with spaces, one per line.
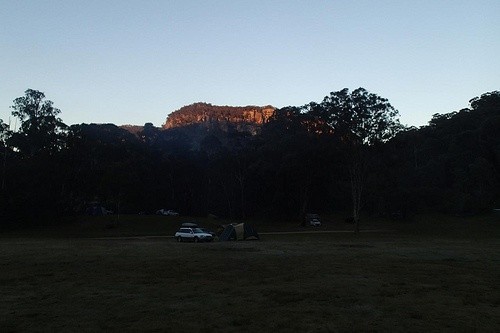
181 223 200 228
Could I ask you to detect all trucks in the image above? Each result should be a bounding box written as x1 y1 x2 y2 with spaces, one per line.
305 213 321 226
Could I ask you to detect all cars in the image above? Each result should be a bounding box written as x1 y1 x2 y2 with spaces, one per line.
164 210 179 216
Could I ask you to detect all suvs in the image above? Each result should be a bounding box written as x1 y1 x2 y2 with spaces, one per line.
174 228 213 242
